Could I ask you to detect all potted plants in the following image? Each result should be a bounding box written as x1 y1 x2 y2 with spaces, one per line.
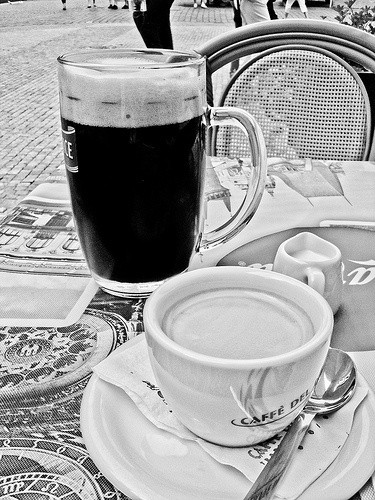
319 1 375 131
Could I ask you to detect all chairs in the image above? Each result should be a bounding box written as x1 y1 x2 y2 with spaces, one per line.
198 20 375 163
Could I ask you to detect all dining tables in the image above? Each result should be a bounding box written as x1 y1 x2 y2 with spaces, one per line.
0 156 373 500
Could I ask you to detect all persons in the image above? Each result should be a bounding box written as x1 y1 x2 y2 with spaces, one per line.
108 0 119 9
121 0 130 9
87 0 97 8
61 0 67 10
193 0 209 9
283 0 309 19
228 0 277 29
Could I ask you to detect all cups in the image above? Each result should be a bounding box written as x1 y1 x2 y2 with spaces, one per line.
58 47 265 296
148 264 338 447
274 232 346 318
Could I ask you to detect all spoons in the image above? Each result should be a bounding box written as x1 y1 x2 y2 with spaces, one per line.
243 348 356 500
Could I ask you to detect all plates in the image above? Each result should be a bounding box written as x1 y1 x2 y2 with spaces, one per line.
78 334 373 499
